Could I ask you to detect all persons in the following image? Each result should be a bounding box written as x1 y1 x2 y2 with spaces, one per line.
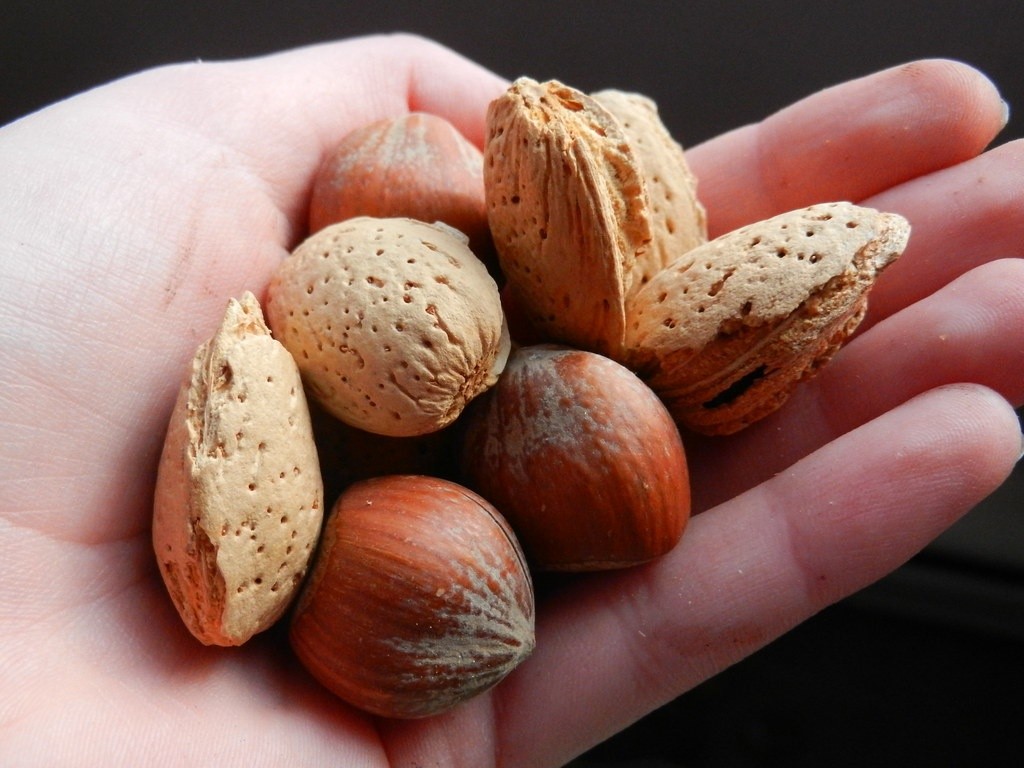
0 32 1024 768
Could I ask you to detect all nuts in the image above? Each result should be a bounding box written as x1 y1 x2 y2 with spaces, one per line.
149 76 911 718
